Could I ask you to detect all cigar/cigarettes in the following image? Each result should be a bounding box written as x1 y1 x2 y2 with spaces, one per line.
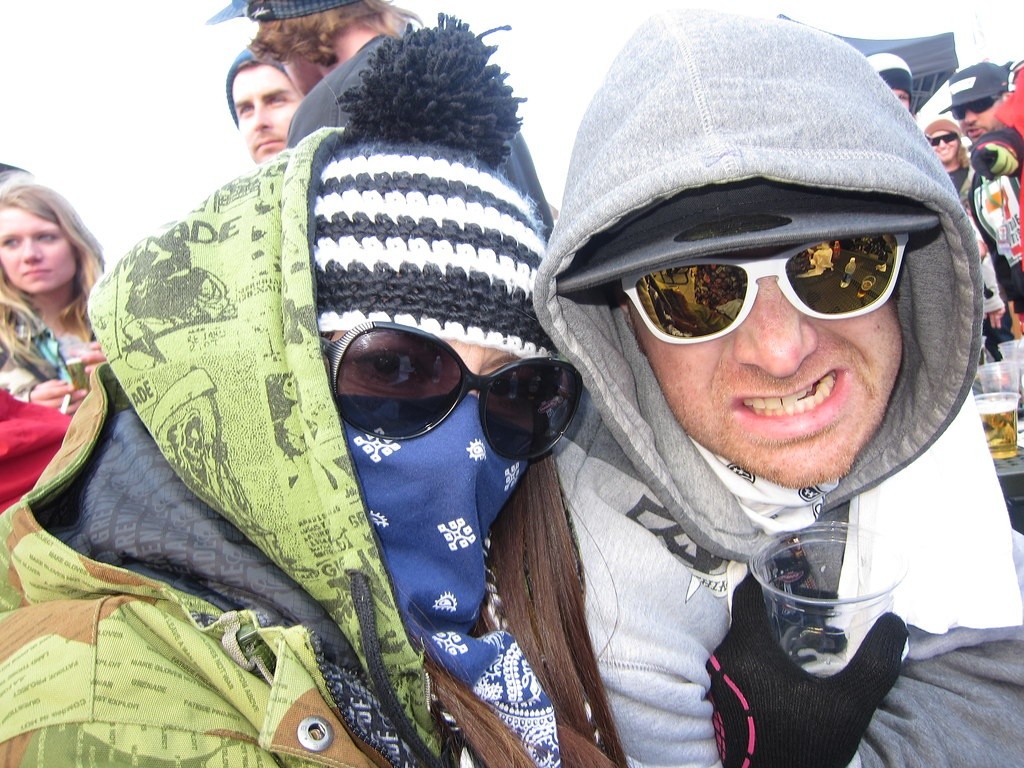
59 394 71 415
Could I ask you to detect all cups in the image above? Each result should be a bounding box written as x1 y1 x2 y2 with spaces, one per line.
748 520 906 679
976 360 1019 401
59 348 90 391
973 393 1020 460
856 276 877 298
998 339 1024 395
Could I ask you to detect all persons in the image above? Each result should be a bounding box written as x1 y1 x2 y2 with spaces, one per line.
225 43 303 167
868 53 913 112
939 62 1024 338
0 15 629 768
654 265 745 335
204 0 554 242
925 119 1014 363
0 181 106 514
534 9 1024 768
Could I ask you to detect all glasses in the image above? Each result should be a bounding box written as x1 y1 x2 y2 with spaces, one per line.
623 218 910 346
927 133 958 146
950 94 1000 120
323 322 588 459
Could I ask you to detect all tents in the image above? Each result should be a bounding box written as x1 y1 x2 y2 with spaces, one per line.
778 13 959 115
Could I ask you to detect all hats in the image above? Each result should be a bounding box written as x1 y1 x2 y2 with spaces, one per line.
939 62 1007 115
225 45 290 126
313 10 572 361
865 52 913 103
553 178 943 306
200 0 383 27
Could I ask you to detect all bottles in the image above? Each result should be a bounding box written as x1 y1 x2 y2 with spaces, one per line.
833 241 842 261
431 355 442 383
840 257 857 288
508 371 518 400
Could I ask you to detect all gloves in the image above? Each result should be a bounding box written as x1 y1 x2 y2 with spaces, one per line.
973 140 1021 182
705 531 910 768
